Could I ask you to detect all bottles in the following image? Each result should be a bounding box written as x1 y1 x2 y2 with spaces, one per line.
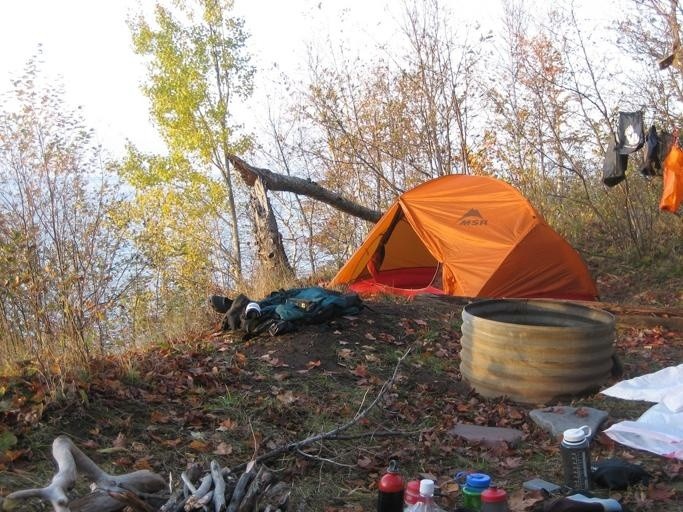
559 425 592 496
376 457 442 511
455 470 492 512
482 483 506 512
244 301 261 319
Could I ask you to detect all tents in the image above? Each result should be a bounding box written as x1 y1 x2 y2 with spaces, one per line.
325 174 600 302
